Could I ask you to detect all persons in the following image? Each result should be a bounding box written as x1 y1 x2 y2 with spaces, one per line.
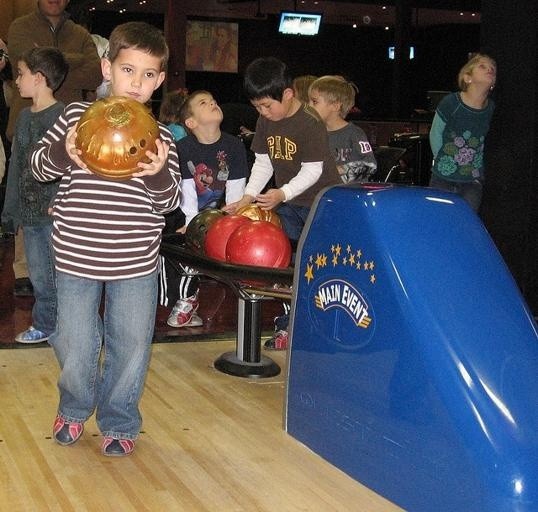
13 48 71 345
31 21 184 458
427 53 496 218
2 0 101 300
0 38 8 184
158 57 377 350
68 13 111 99
186 22 234 72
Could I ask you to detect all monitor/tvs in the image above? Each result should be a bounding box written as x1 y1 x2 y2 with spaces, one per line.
389 46 415 62
279 11 322 37
